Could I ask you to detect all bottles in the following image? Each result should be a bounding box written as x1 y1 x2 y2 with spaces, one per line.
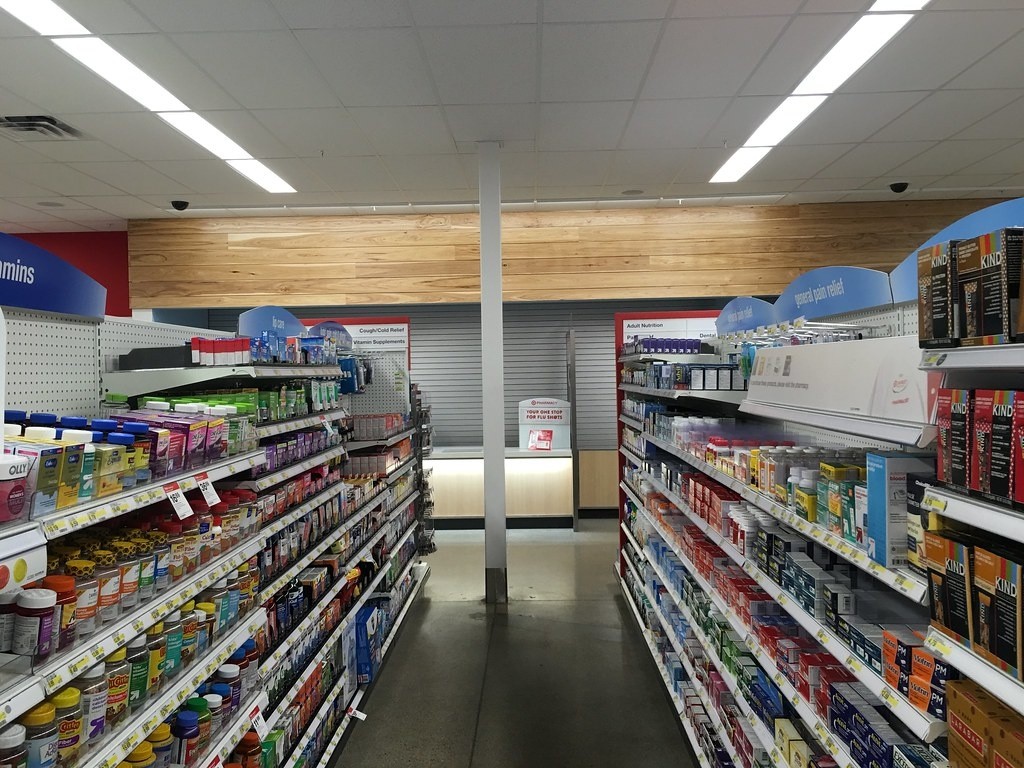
672 415 879 557
0 383 313 768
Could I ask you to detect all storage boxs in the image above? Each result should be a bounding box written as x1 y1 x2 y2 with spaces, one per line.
1 333 421 768
615 226 1022 768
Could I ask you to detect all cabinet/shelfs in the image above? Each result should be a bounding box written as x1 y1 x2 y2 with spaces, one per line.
0 306 266 768
611 310 748 639
347 426 421 669
100 311 368 768
916 304 1024 768
643 305 947 768
